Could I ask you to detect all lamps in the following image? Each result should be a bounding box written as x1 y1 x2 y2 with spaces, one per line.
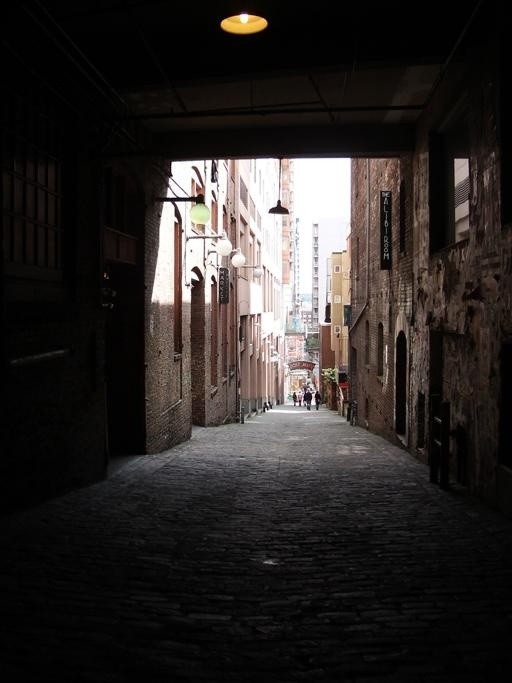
154 194 262 278
268 156 290 215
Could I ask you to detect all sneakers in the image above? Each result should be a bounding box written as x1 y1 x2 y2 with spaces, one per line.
294 406 296 407
309 408 310 410
299 405 301 406
316 408 318 410
307 408 309 409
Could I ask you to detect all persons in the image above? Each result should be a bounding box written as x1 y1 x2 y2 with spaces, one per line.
292 390 297 406
303 389 312 410
314 390 321 411
297 389 303 407
